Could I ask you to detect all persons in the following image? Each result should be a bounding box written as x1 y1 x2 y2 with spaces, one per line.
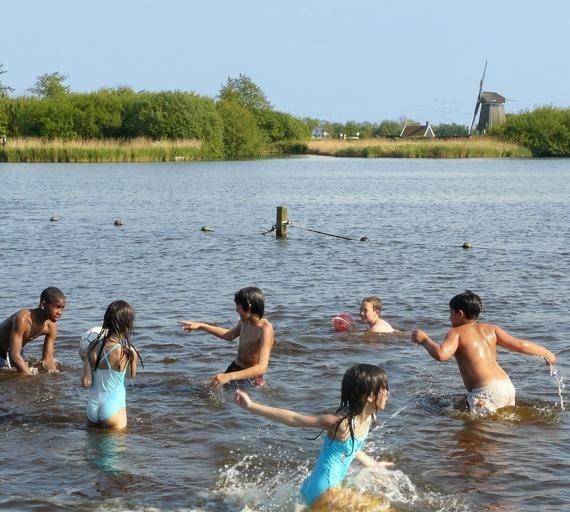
0 287 66 375
411 291 556 419
360 297 395 334
81 300 144 431
176 287 272 391
234 364 394 512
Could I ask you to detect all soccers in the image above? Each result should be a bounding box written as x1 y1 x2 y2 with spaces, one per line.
333 313 353 331
79 327 108 350
